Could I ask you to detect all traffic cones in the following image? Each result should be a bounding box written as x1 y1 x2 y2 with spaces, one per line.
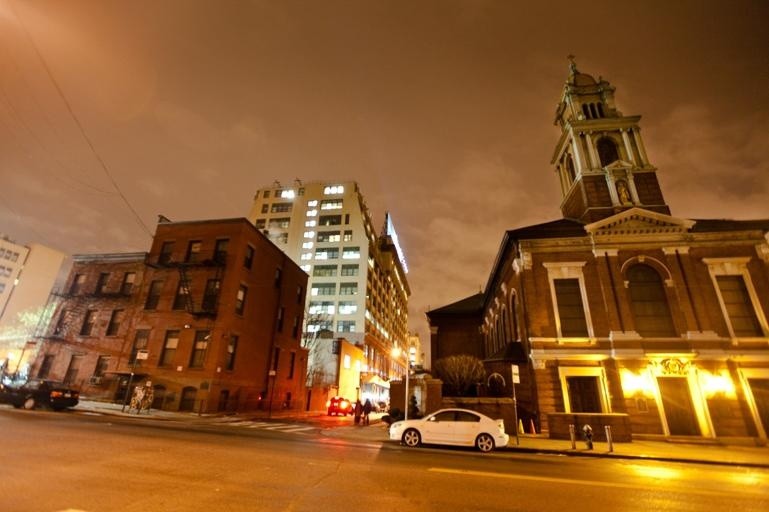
518 419 524 435
529 419 537 434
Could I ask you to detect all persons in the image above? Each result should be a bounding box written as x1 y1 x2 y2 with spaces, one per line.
0 357 9 385
20 362 30 381
362 398 371 425
354 400 364 423
137 381 154 415
126 384 146 414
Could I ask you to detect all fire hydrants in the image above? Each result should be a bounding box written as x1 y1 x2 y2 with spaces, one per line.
583 425 596 450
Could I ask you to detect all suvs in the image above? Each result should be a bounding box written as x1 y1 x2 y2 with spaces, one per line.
327 397 352 416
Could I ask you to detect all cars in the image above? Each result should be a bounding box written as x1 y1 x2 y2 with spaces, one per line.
375 401 386 413
1 376 80 411
388 407 509 452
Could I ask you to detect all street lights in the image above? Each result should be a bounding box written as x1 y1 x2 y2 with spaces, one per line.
390 344 417 420
